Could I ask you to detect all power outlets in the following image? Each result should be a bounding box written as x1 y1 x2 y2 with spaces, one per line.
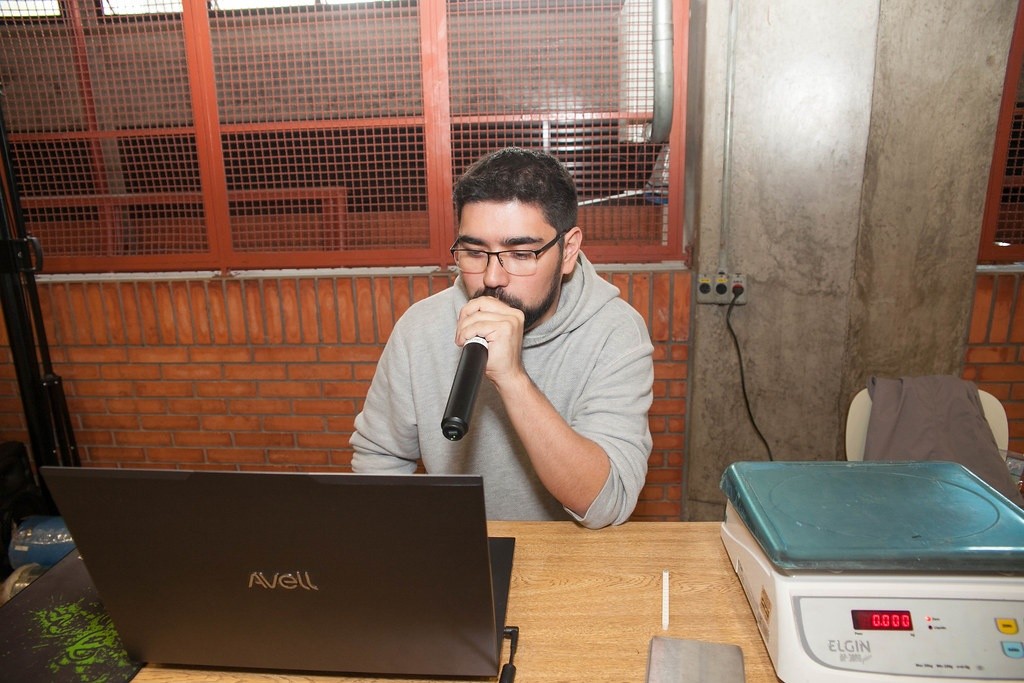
696 273 748 305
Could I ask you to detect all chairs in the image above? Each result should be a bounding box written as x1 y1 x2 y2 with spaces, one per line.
842 387 1008 465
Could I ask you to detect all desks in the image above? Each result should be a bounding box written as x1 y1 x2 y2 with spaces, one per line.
0 522 782 682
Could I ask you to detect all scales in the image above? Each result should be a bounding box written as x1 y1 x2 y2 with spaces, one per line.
721 459 1024 682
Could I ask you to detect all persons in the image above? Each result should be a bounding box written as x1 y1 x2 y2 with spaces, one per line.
347 143 657 531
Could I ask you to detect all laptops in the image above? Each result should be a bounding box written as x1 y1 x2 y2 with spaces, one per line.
37 465 515 677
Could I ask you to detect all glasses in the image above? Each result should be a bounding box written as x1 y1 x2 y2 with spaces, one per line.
450 229 568 276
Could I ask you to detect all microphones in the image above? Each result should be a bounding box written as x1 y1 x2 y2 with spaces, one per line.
441 334 489 441
